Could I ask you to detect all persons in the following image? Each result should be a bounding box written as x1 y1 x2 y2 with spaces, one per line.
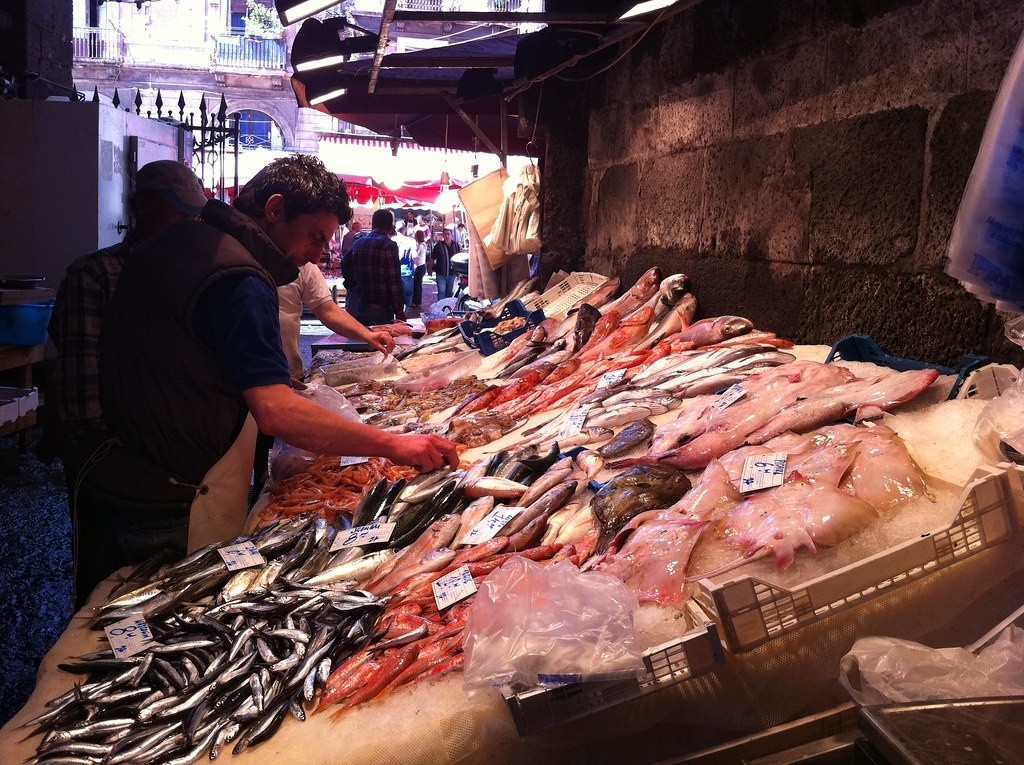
73 153 459 611
32 159 465 517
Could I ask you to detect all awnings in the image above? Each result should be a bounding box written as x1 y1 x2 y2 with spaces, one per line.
285 0 689 156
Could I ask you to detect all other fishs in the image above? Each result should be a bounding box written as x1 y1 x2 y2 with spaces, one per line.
340 267 939 602
11 477 580 765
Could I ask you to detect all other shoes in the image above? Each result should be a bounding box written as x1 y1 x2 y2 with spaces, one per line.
410 302 421 307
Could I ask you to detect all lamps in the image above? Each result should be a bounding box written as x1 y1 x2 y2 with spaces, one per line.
270 75 283 90
102 64 121 80
215 71 226 87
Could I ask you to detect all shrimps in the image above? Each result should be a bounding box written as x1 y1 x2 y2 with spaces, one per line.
259 452 419 523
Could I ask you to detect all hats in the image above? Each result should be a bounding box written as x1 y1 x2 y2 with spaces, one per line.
132 160 209 207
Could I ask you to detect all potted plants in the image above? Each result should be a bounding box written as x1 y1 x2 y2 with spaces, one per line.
443 1 460 33
398 1 409 29
